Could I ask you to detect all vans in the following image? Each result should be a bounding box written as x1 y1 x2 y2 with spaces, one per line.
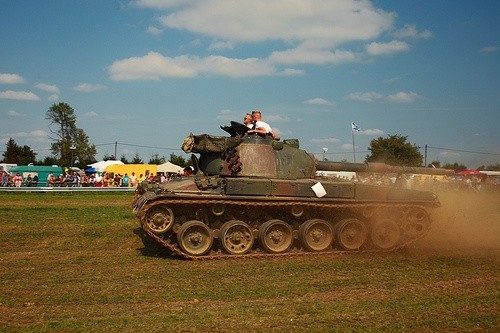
0 170 13 187
101 163 157 187
8 165 64 187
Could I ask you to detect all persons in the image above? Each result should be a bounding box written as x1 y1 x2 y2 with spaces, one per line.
12 173 23 187
338 174 499 193
243 111 280 141
25 174 32 187
32 175 38 187
47 170 184 187
1 172 7 187
5 173 12 187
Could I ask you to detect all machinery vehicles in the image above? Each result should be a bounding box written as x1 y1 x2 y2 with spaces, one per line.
131 121 456 261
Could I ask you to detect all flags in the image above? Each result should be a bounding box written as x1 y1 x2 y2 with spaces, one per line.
352 122 360 132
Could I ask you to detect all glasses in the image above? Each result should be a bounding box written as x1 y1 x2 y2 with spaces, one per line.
244 118 247 120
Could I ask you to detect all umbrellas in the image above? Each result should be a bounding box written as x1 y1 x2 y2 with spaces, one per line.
69 160 125 175
153 162 187 176
454 170 484 177
